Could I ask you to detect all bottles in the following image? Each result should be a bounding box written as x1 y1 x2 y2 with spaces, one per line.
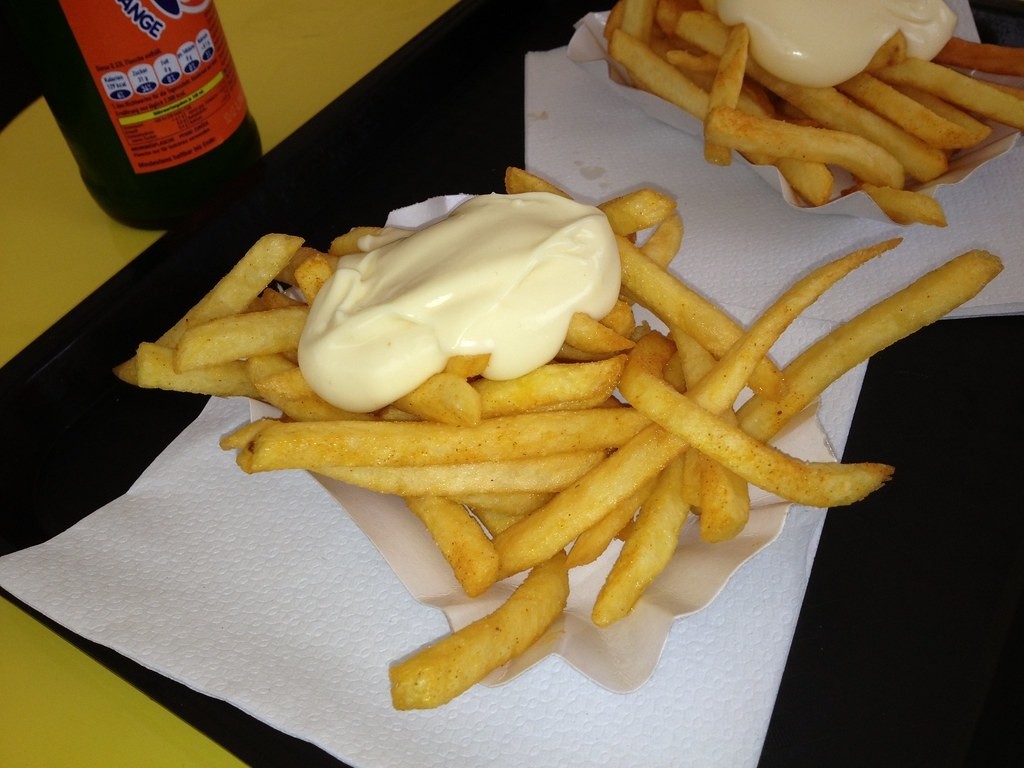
2 0 262 231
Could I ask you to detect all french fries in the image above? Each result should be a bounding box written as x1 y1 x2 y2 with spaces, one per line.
112 161 1006 712
601 0 1024 228
